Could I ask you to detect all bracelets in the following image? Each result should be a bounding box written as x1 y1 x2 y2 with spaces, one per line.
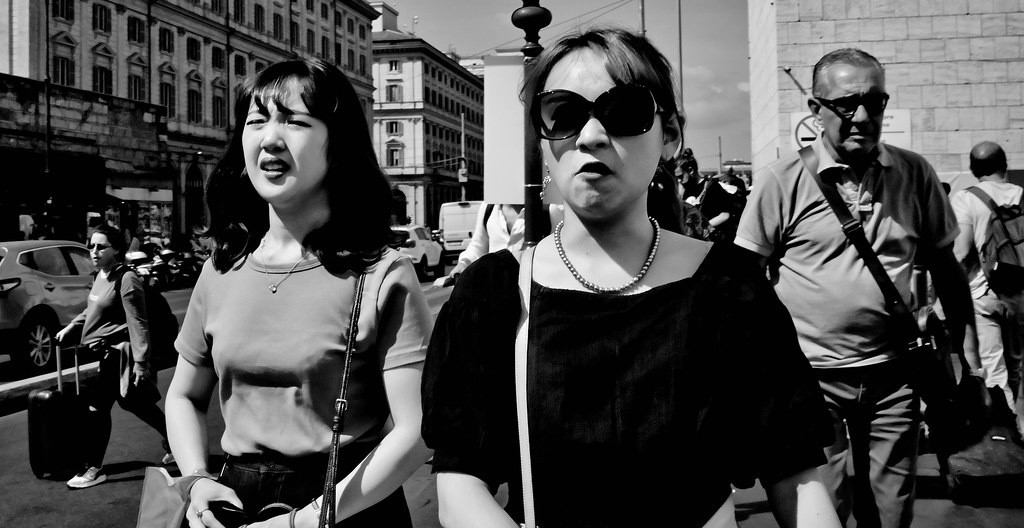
290 508 300 528
311 499 331 528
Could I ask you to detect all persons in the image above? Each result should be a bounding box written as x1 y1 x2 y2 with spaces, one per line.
165 60 437 528
411 30 1024 528
55 225 177 489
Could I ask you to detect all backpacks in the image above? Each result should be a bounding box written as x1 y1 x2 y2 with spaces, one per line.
963 187 1024 326
113 267 179 373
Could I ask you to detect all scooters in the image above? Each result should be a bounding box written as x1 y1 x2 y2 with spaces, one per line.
124 248 185 293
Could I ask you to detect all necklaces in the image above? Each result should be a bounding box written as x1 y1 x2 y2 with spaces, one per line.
553 216 662 293
260 244 313 292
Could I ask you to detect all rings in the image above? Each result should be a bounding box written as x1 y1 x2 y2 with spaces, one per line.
195 508 211 518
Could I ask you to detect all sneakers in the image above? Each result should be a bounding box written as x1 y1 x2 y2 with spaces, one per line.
66 463 108 489
158 452 178 466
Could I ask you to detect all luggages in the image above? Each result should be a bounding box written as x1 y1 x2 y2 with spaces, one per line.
27 331 89 480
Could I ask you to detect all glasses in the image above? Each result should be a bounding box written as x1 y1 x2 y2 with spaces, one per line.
530 82 667 141
87 243 112 251
675 174 683 184
817 91 889 120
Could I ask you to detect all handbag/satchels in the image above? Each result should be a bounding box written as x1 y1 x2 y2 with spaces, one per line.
910 307 957 407
137 465 220 528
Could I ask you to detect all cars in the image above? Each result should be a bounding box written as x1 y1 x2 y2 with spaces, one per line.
0 239 179 374
